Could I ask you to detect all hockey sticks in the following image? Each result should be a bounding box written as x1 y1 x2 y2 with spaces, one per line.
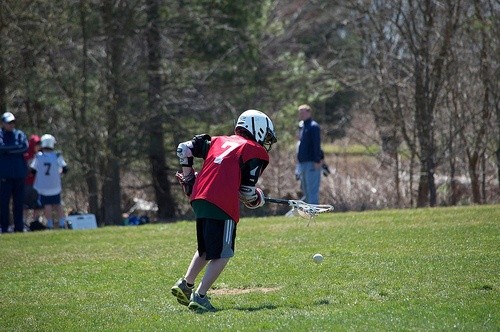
264 197 334 220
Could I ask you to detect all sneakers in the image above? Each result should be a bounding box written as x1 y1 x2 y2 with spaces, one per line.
188 289 215 311
171 275 195 307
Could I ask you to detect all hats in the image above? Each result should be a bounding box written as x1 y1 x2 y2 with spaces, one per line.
2 112 15 123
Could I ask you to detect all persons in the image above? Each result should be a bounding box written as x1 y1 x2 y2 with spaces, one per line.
0 112 28 234
22 135 41 232
171 110 277 312
30 134 67 229
294 104 330 205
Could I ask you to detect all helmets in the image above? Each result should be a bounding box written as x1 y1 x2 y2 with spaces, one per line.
41 134 57 149
235 109 277 144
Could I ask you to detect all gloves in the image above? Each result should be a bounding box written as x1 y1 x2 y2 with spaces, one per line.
175 167 198 199
244 188 266 209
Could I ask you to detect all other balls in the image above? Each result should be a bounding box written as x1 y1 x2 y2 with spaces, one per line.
313 254 323 264
